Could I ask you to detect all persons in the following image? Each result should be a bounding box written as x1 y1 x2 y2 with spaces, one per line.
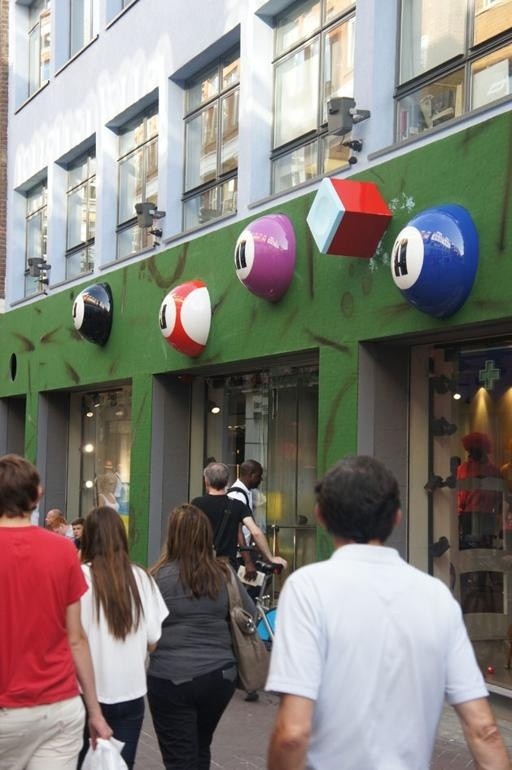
144 501 262 770
261 453 511 770
70 504 169 768
190 457 291 702
454 430 500 610
224 458 265 629
44 508 69 538
0 451 116 769
69 516 88 563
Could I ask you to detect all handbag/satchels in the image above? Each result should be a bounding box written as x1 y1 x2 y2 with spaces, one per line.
222 561 275 694
80 735 130 770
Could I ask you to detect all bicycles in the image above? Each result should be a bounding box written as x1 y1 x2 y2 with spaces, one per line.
233 546 284 653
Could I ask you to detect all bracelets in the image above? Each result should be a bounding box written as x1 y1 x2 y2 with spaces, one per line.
244 560 250 564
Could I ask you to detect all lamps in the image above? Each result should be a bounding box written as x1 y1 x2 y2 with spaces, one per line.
325 96 371 136
25 256 53 277
135 201 168 230
